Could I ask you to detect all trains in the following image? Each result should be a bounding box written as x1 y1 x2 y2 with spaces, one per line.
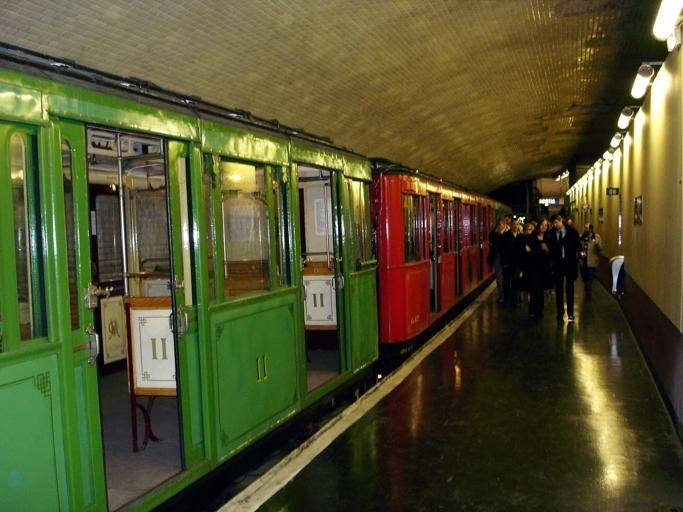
1 40 516 512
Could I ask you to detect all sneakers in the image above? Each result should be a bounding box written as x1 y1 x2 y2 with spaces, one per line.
555 313 564 320
567 314 575 320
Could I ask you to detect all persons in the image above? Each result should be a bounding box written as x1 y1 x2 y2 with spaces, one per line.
486 212 607 322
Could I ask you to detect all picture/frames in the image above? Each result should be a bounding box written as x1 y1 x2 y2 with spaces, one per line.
632 194 643 228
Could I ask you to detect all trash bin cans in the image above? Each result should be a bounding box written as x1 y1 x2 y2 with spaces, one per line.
608 254 625 297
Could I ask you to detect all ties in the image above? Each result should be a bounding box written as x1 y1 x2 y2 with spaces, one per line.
558 231 563 240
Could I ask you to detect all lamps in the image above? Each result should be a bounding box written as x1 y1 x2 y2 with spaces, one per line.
651 0 683 42
617 106 634 130
610 131 623 149
630 64 654 99
